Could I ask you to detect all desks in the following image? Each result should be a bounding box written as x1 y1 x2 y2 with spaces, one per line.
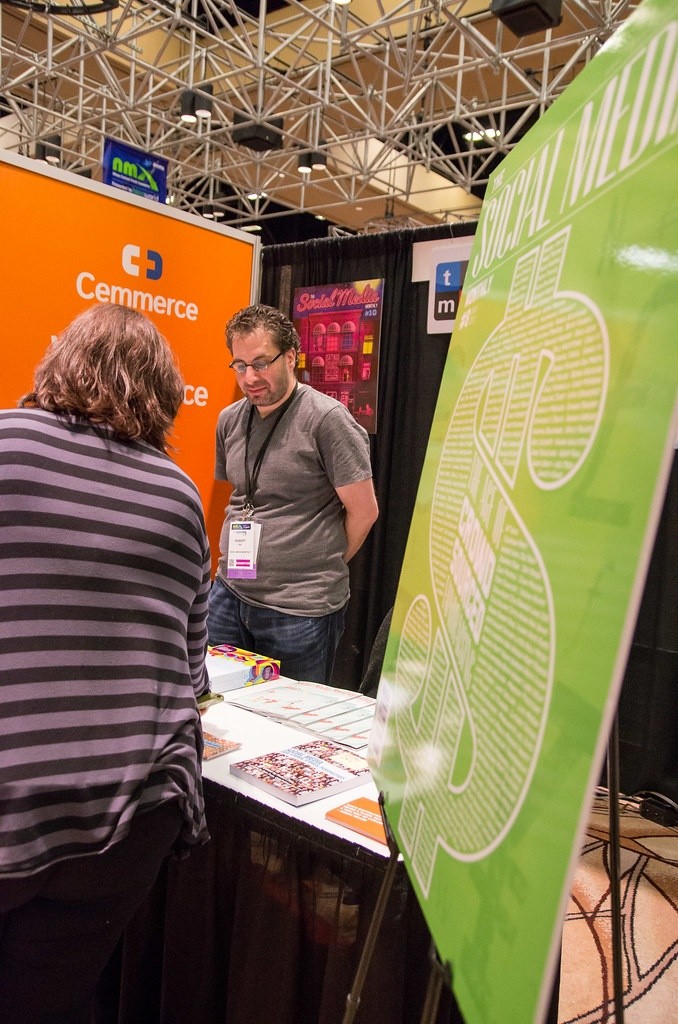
117 645 563 1024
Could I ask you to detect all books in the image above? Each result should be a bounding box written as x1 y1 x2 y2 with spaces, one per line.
195 643 389 848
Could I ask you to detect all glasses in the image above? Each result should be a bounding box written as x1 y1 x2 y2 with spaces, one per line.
229 350 284 374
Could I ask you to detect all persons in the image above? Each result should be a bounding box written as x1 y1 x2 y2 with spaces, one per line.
205 304 378 685
0 302 208 1024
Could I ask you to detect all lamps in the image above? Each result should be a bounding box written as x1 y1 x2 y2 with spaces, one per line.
179 83 214 123
298 139 327 173
34 134 61 164
